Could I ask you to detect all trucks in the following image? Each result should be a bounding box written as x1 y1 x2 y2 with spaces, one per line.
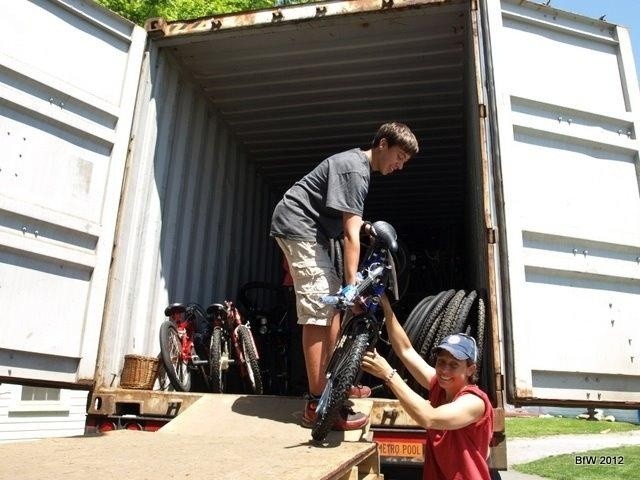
1 1 640 480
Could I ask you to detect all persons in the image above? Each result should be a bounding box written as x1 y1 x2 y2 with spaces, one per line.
266 120 421 430
354 286 494 479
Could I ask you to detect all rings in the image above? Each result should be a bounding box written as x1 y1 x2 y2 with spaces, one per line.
372 353 377 359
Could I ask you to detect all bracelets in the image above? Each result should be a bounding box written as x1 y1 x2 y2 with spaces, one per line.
385 368 397 387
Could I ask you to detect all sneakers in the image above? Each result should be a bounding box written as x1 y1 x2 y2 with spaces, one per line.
348 383 372 399
301 392 369 431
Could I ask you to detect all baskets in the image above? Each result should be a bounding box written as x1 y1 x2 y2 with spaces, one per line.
120 355 160 390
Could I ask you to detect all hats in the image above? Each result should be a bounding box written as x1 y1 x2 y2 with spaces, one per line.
431 333 479 364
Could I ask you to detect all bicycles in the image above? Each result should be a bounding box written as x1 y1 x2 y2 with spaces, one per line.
240 233 464 395
154 295 271 399
302 220 404 444
